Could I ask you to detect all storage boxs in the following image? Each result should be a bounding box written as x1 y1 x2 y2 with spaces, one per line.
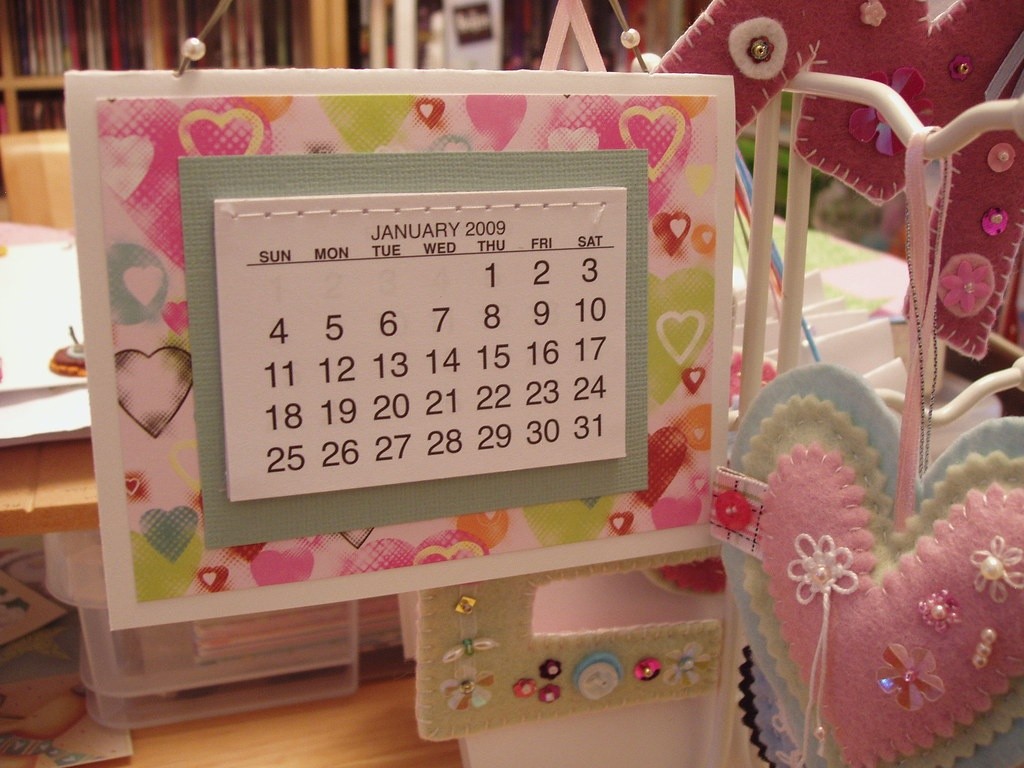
40 530 405 728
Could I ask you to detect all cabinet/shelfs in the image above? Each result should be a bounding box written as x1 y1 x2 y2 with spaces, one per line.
0 0 349 134
